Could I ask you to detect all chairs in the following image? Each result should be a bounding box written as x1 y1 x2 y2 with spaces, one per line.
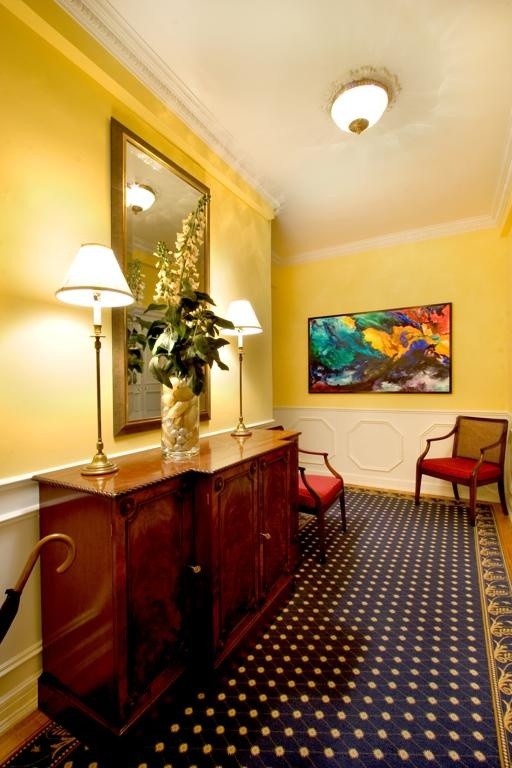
265 426 347 564
414 416 509 527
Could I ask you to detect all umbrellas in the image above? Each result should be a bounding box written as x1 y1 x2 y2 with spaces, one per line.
1 533 77 643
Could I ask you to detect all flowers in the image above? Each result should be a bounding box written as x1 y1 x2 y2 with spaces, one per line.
138 192 236 396
125 256 148 385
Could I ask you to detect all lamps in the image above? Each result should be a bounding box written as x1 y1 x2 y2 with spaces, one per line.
219 300 264 437
307 302 453 395
323 65 402 137
124 182 156 214
54 242 137 478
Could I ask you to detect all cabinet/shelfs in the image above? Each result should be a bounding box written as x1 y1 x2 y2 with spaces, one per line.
33 430 302 759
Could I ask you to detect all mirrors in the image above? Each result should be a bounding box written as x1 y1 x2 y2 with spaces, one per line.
109 115 213 440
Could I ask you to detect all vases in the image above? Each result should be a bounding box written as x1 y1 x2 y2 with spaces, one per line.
160 376 201 462
